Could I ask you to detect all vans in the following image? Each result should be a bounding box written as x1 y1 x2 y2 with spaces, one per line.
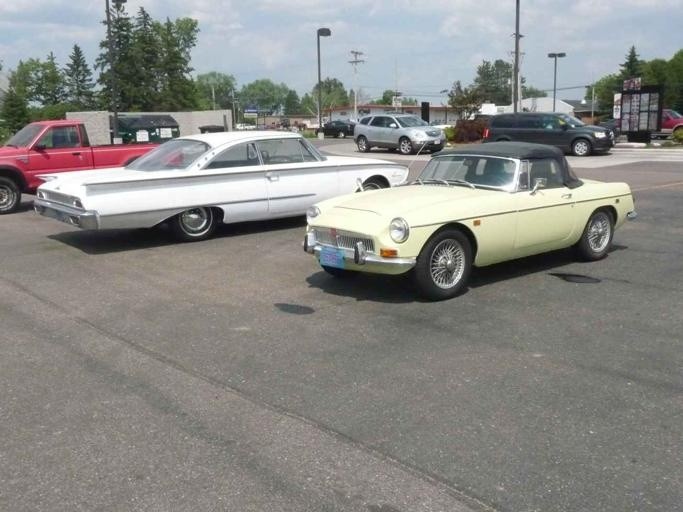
483 112 615 160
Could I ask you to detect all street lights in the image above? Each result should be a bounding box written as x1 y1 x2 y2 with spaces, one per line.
105 0 125 140
315 27 332 128
585 86 595 125
547 53 566 112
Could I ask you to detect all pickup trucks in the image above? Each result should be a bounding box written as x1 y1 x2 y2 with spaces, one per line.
0 117 184 214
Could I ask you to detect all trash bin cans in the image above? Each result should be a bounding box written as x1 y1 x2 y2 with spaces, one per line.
200 126 225 133
299 124 305 130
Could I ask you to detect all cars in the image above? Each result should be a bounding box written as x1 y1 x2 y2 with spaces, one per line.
314 120 356 138
650 108 683 140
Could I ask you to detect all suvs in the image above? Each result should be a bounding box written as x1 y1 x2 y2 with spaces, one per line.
236 123 257 131
352 112 446 155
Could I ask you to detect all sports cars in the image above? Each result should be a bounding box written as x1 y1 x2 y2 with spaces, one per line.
32 130 411 242
304 139 639 299
